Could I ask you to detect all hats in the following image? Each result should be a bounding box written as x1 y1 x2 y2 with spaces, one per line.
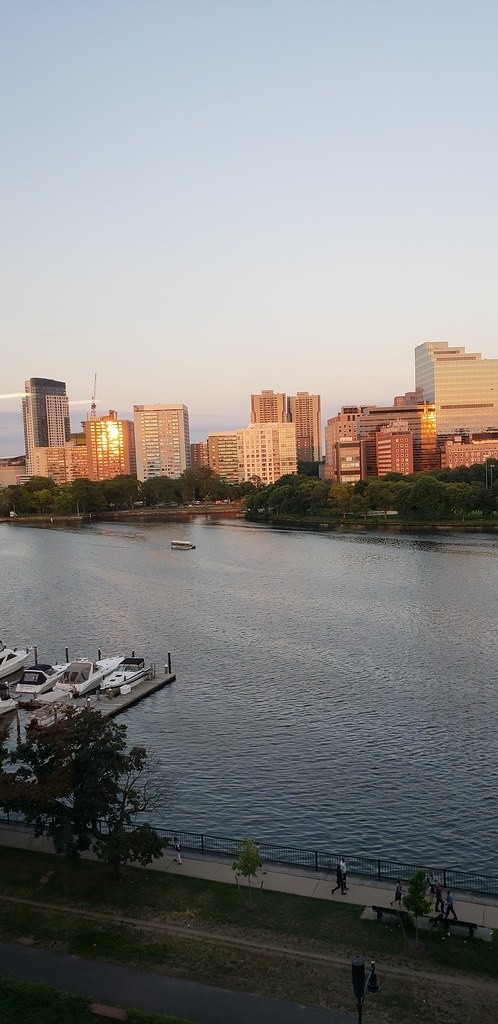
342 858 345 862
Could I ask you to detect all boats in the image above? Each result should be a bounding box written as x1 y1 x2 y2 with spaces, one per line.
0 639 32 677
10 658 72 695
169 540 196 551
101 648 152 695
56 655 122 695
0 682 20 715
28 700 73 729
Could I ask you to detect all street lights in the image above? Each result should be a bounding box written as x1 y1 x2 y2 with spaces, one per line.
351 957 381 1024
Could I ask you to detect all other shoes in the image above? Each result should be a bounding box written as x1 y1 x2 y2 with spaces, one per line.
341 893 347 895
390 903 393 906
435 910 441 912
344 887 349 890
399 907 402 909
441 911 446 914
332 889 334 894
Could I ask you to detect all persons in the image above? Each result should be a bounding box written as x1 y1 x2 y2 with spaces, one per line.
445 893 458 920
172 836 183 865
331 864 347 895
428 874 447 914
340 857 349 890
390 880 406 908
